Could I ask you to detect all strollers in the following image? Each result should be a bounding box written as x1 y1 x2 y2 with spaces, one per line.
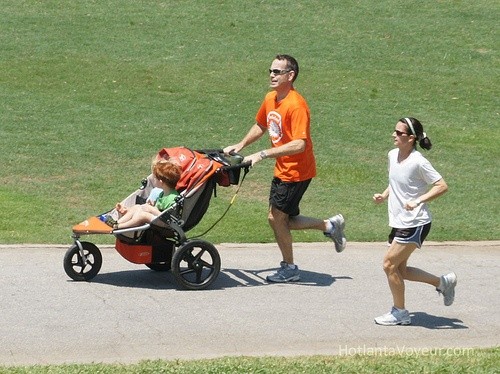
63 145 253 291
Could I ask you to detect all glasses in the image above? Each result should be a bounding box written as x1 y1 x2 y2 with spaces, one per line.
394 130 408 136
269 69 293 75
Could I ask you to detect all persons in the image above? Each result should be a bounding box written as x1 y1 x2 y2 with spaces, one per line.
222 54 347 284
104 160 181 230
372 116 458 327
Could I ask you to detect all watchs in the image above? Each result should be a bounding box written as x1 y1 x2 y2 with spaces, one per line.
259 151 267 159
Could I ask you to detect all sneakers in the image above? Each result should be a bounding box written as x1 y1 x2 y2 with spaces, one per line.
374 305 411 326
435 271 458 306
323 214 346 253
266 262 302 283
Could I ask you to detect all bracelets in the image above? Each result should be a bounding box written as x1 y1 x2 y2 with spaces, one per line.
415 199 420 207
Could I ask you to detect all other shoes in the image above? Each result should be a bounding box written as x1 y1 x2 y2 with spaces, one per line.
104 218 115 228
105 215 117 224
